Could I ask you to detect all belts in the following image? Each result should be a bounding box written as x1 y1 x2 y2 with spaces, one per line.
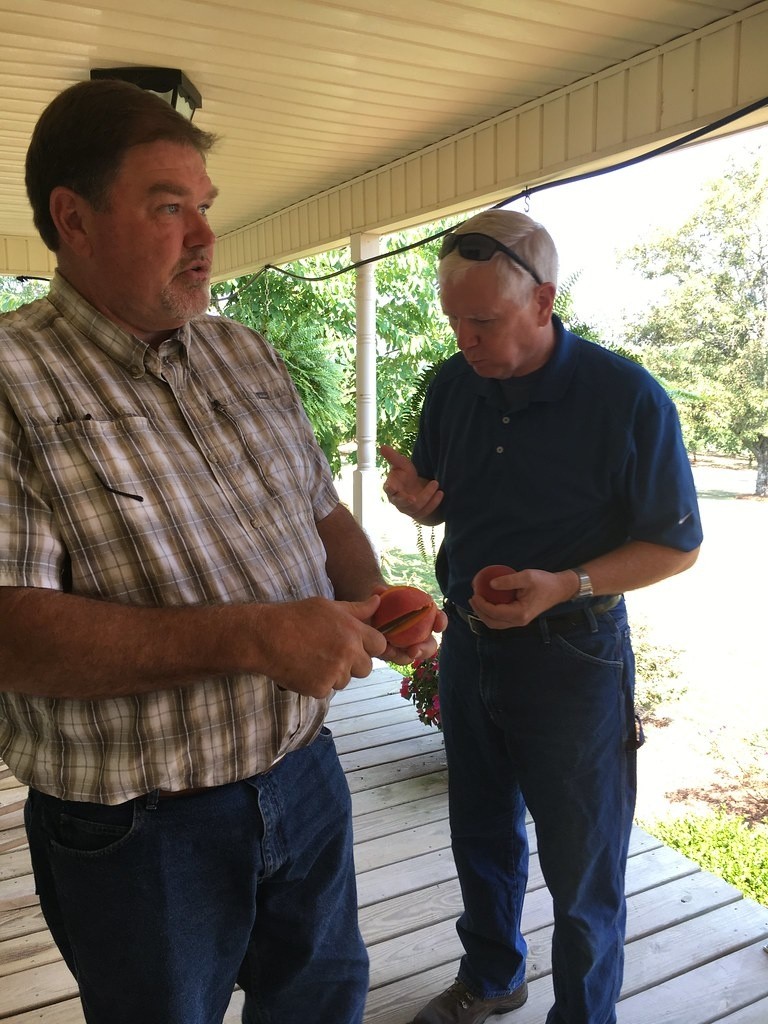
449 594 622 638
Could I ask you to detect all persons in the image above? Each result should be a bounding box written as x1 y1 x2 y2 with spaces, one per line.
0 80 447 1023
379 210 704 1024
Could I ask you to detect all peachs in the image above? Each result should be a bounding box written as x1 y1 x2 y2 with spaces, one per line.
371 587 437 647
473 565 519 603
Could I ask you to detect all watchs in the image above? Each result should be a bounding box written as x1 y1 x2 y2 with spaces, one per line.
569 567 592 606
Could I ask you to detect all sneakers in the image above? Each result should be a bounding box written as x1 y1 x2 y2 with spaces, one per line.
413 980 527 1024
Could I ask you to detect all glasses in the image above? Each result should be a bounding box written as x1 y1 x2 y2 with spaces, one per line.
438 232 543 286
